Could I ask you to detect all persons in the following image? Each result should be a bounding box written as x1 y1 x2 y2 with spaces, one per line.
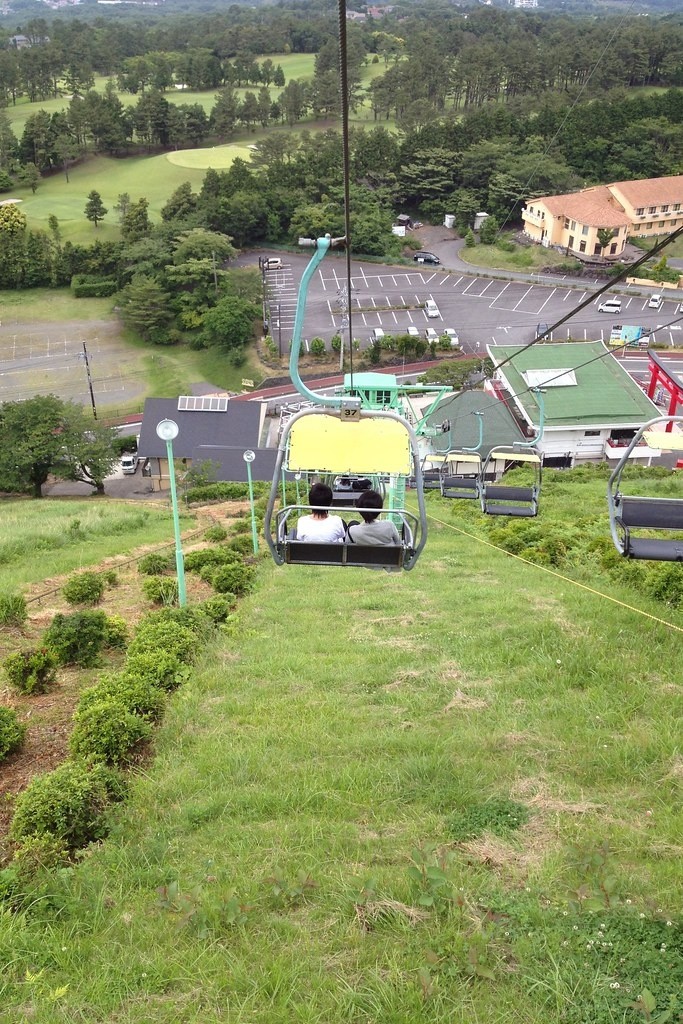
348 491 405 547
334 476 374 493
291 484 347 543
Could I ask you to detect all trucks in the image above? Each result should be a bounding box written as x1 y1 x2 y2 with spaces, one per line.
120 456 140 474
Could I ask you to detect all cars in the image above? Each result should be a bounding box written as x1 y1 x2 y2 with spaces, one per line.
443 327 459 346
413 251 440 266
406 327 419 339
535 322 549 342
426 328 439 345
424 299 440 318
373 327 385 340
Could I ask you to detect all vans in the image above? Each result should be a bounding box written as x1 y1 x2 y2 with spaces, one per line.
260 256 283 270
648 294 663 308
598 300 622 314
